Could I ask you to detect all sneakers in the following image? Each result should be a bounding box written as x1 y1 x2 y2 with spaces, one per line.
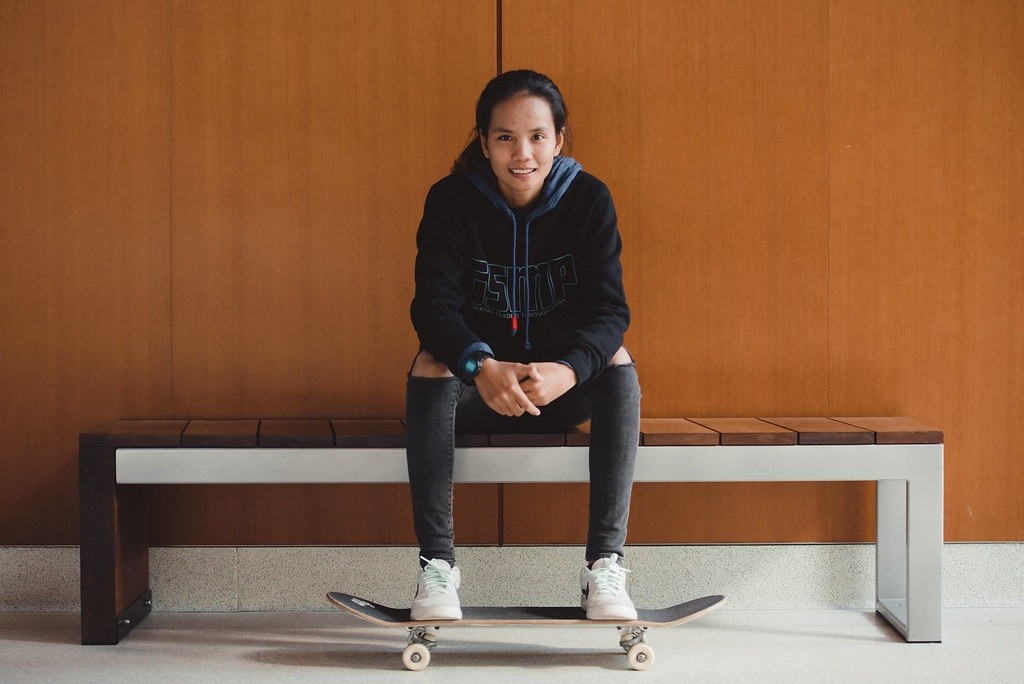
578 553 638 621
410 555 463 620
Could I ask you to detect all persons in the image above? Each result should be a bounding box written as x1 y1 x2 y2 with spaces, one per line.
406 69 642 620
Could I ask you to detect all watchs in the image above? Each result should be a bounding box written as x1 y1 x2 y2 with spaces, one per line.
464 351 494 386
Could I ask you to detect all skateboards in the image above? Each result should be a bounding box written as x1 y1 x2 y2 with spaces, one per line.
324 590 728 671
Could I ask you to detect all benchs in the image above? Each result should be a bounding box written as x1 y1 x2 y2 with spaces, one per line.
78 417 945 645
